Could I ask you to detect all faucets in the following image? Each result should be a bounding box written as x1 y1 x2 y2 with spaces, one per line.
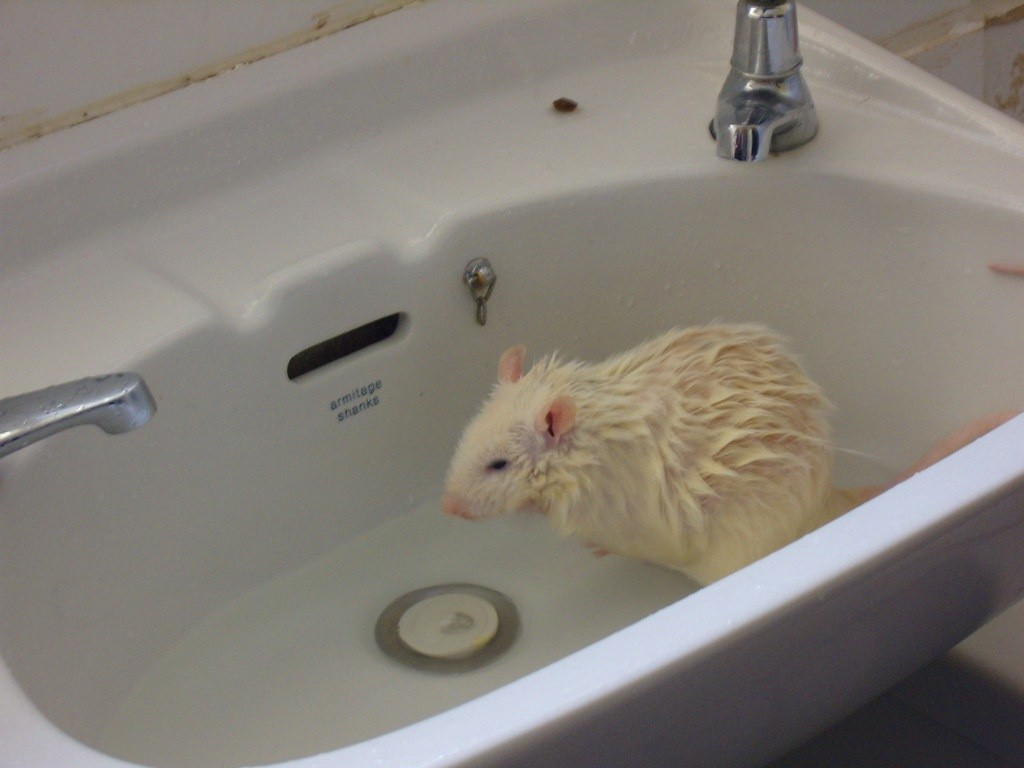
0 371 158 459
709 0 820 165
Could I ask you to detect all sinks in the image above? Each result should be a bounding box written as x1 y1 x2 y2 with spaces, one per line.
0 158 1024 767
